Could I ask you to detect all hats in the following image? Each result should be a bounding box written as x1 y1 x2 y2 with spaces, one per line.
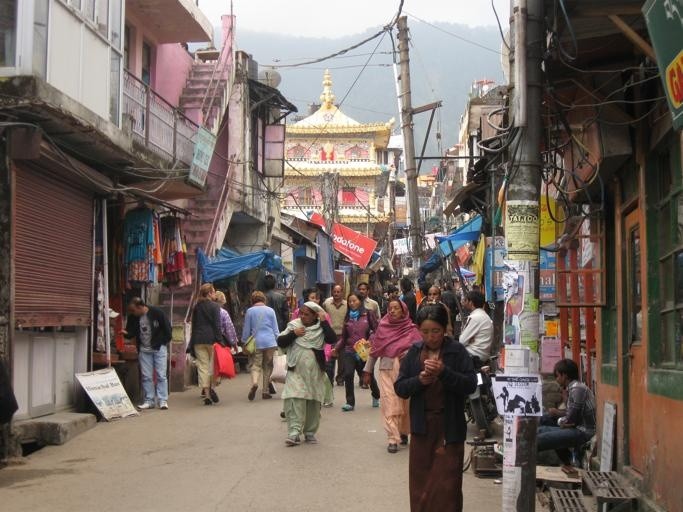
388 284 399 296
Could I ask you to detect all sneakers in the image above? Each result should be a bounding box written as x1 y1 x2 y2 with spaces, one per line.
360 382 369 388
399 438 409 446
336 379 344 385
137 402 154 408
343 403 353 410
373 397 379 407
387 444 397 451
201 389 219 404
285 433 318 444
159 399 168 408
249 382 276 400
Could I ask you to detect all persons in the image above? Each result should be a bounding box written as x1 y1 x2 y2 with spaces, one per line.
393 300 477 510
186 272 496 454
532 353 597 489
539 386 574 465
117 294 173 410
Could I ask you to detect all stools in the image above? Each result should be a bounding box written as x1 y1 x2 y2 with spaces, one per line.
568 440 596 471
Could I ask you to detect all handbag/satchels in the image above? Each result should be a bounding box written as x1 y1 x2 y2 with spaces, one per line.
242 336 257 356
213 342 236 379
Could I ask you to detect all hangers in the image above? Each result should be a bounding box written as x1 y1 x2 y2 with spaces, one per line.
130 198 177 218
